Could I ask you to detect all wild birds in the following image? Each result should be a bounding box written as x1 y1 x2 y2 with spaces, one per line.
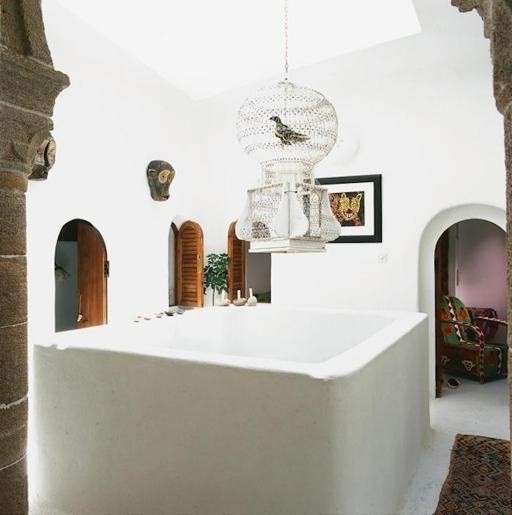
268 116 312 146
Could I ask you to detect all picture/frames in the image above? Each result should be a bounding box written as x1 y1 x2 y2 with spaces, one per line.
315 175 382 243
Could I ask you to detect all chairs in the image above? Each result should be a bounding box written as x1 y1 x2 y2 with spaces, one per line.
440 296 508 383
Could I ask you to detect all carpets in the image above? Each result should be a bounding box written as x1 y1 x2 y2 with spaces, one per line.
433 434 512 515
